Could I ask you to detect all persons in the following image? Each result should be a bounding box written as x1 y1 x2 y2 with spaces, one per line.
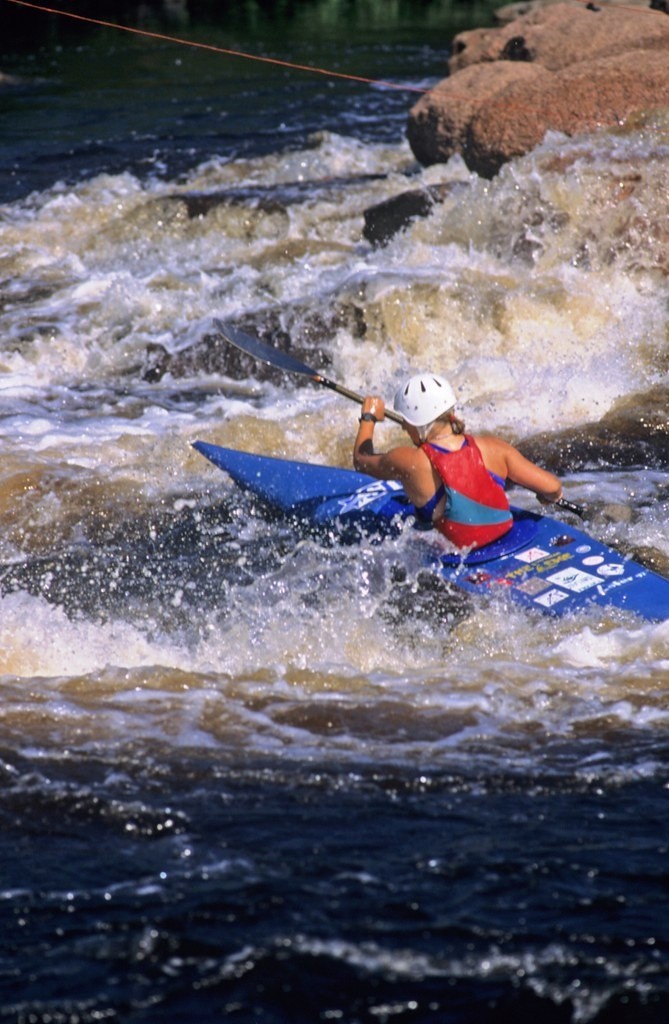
353 372 563 554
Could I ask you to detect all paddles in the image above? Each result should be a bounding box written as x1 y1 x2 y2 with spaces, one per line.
209 312 642 532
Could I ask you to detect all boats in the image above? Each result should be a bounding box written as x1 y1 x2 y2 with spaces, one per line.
192 436 668 633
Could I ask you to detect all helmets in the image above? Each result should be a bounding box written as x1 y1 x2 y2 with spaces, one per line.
393 373 458 427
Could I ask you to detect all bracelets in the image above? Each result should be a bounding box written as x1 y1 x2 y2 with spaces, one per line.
358 413 377 424
